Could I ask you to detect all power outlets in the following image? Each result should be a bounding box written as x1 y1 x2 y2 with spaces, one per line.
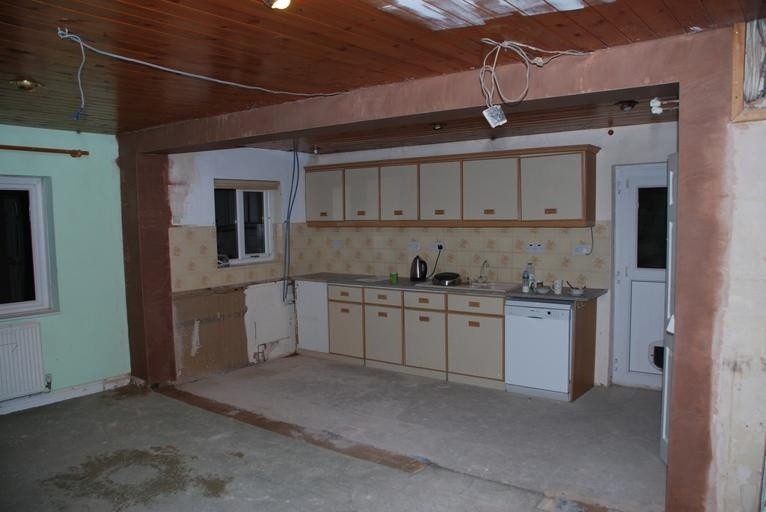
436 242 445 253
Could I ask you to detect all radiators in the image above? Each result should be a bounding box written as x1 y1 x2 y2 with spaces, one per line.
0 321 51 402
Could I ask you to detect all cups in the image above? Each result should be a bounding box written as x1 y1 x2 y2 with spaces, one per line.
390 272 398 286
550 279 563 295
567 287 585 296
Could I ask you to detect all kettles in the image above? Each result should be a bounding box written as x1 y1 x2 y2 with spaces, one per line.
410 255 427 282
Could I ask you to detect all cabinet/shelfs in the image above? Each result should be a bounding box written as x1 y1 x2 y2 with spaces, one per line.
305 144 601 228
328 285 504 389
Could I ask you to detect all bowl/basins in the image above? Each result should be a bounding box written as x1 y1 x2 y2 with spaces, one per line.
535 287 550 294
431 272 461 286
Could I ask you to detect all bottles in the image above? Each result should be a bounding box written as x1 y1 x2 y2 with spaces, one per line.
537 279 543 288
521 269 530 293
526 262 535 290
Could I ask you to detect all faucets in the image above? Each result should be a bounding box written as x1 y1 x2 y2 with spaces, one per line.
478 258 490 282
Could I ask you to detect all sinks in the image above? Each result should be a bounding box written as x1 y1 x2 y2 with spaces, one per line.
452 284 495 290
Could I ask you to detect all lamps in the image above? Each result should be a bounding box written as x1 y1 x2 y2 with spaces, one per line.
431 123 443 130
621 101 633 112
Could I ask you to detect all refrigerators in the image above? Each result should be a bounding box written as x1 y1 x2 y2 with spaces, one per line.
502 297 573 403
